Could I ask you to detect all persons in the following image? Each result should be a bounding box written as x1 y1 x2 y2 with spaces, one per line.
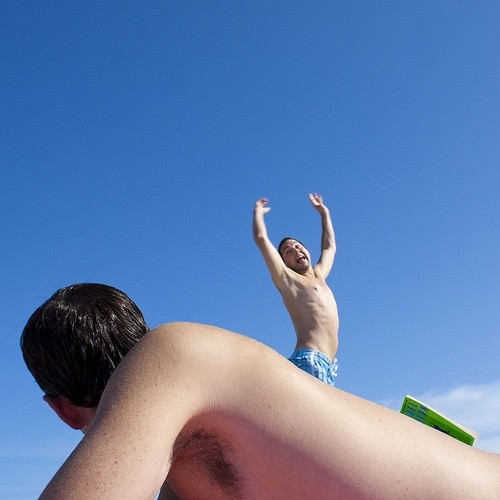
19 283 500 500
251 191 342 385
